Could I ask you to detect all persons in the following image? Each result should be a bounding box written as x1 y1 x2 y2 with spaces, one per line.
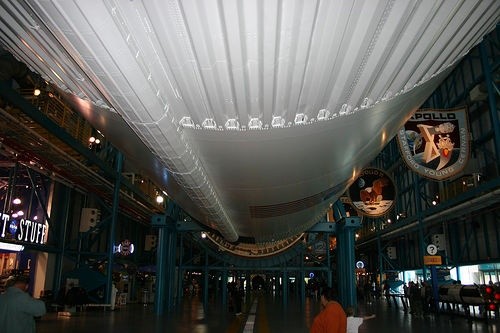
0 276 46 333
310 287 347 333
231 279 245 315
186 279 197 309
346 307 376 333
357 279 431 317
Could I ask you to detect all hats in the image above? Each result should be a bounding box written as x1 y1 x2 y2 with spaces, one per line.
17 274 32 283
